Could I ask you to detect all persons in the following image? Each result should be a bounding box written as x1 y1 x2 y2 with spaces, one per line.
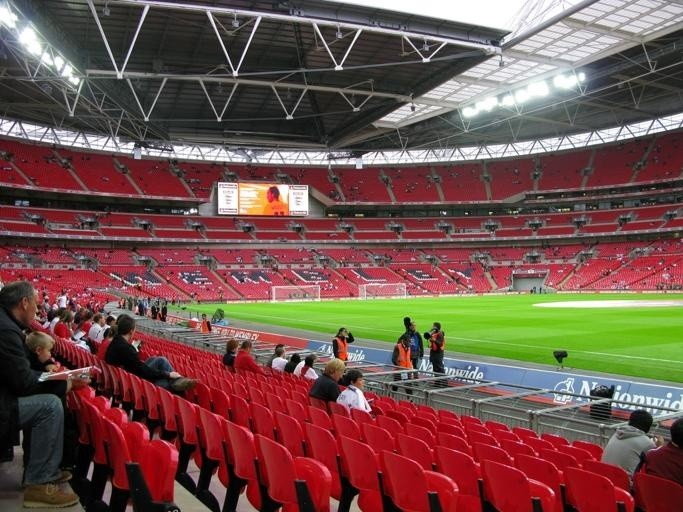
600 409 664 491
645 417 681 486
390 335 412 402
397 322 423 386
428 321 448 388
334 369 372 417
401 316 411 334
1 152 320 508
307 359 345 403
322 137 683 292
331 327 354 363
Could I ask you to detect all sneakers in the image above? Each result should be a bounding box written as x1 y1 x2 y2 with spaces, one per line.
172 377 199 391
45 470 72 483
23 483 80 507
